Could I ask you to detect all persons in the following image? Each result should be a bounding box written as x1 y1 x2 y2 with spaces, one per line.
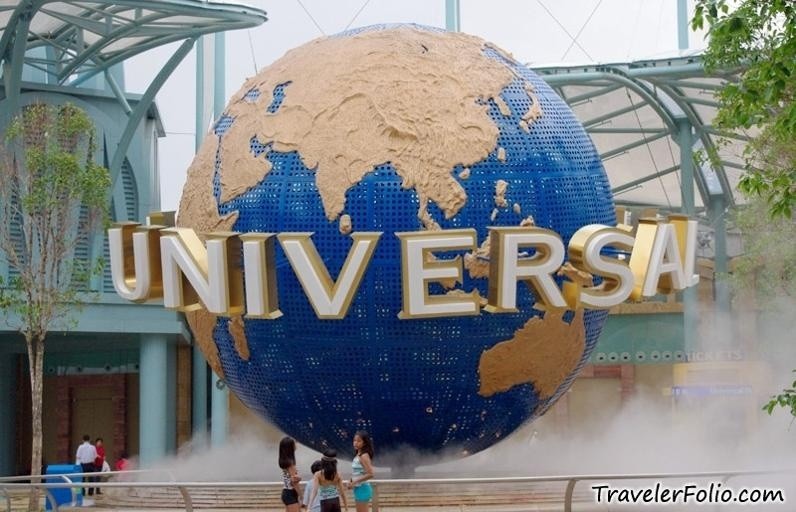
114 452 133 471
278 437 304 512
300 459 321 512
76 434 97 496
91 437 105 494
306 455 349 512
347 430 375 512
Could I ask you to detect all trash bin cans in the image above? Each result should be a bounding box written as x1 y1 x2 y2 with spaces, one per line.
45 464 83 512
